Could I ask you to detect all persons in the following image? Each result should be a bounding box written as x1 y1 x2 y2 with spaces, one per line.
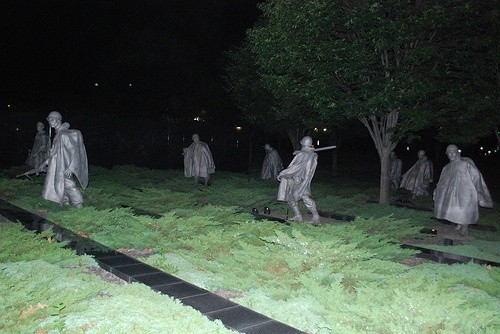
42 112 89 208
277 136 320 225
28 121 51 175
433 144 494 237
183 134 216 187
399 150 434 200
258 143 284 181
